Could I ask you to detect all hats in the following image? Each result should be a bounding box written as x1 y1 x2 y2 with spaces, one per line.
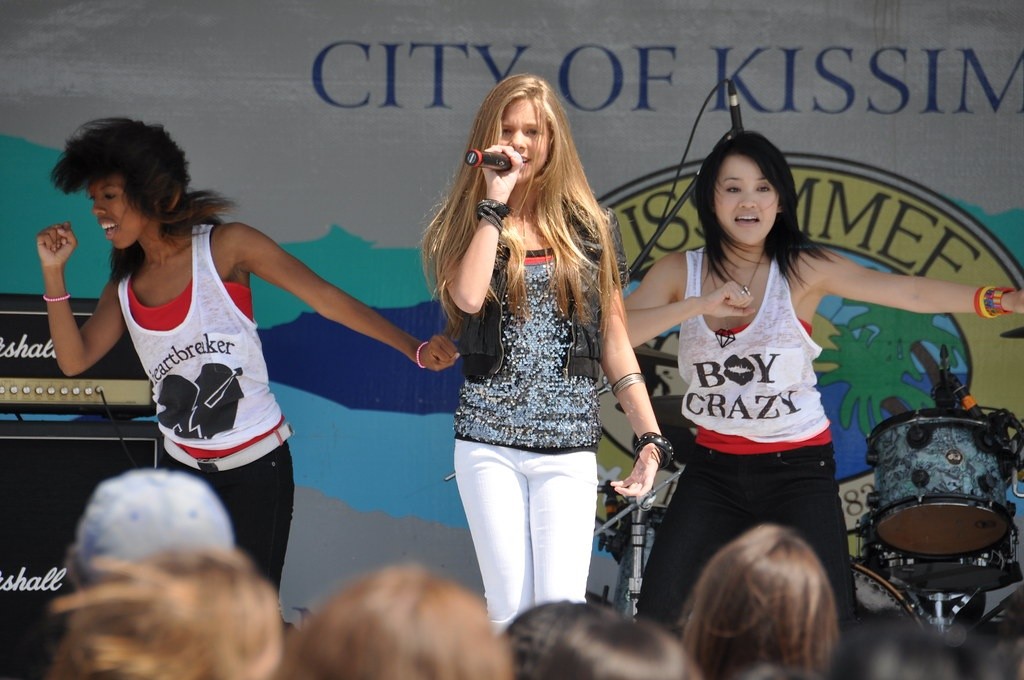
76 468 234 591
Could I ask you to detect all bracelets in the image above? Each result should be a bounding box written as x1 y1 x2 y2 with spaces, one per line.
972 284 1016 320
633 432 675 471
477 198 509 233
611 373 646 397
42 293 70 302
417 339 429 370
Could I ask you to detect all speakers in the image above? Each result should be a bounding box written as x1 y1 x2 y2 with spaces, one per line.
0 420 160 680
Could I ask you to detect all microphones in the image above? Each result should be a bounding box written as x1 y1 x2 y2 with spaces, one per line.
603 480 619 529
949 373 983 419
940 342 951 390
464 148 513 172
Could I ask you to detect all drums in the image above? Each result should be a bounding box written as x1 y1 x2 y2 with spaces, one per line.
850 561 942 680
876 516 1017 594
867 408 1009 553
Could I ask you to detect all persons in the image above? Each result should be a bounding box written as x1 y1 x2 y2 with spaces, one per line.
293 562 516 679
36 117 460 589
422 71 675 637
833 604 1024 676
682 522 840 680
502 599 701 680
619 128 1024 635
51 466 288 679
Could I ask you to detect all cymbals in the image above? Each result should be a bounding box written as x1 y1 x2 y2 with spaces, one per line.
634 344 677 367
613 394 699 456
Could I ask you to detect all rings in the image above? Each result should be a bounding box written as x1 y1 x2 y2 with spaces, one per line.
741 290 745 295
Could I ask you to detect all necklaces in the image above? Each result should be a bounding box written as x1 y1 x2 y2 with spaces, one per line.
716 248 765 290
